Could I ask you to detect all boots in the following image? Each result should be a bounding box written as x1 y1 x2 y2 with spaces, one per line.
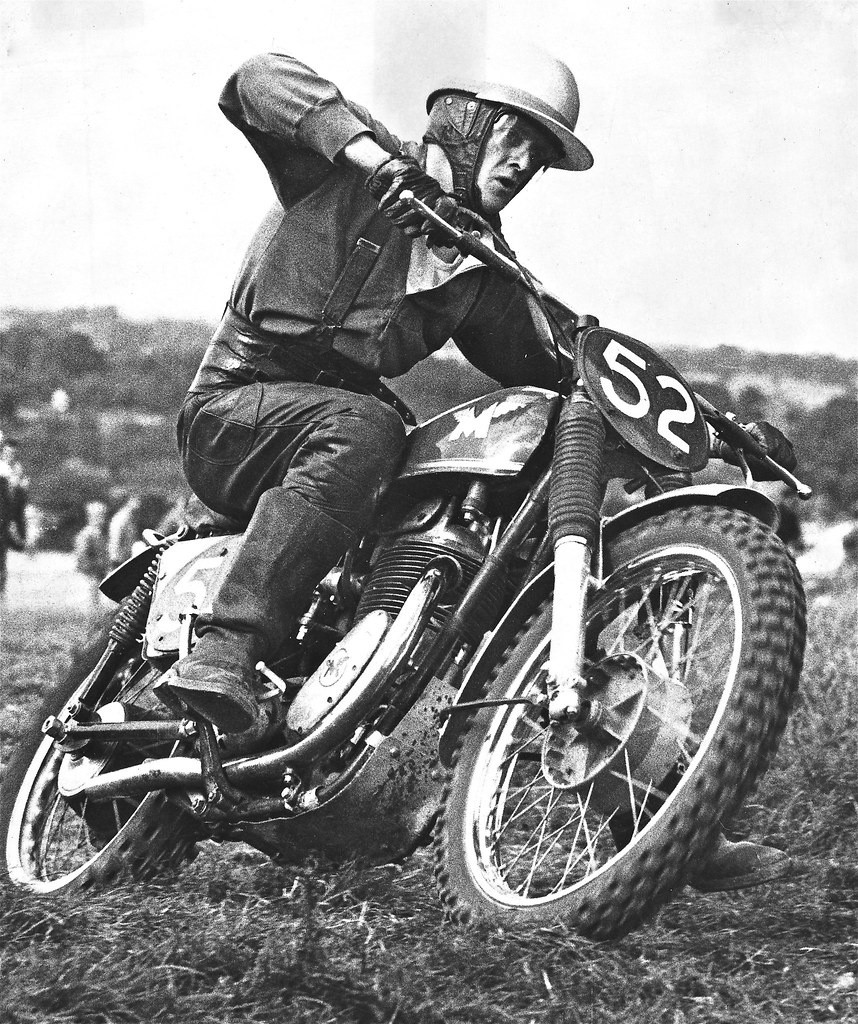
607 623 792 890
151 485 357 733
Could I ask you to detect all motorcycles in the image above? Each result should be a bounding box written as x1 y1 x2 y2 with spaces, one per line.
3 186 814 943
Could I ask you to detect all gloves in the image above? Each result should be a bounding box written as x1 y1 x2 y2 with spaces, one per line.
712 421 797 481
362 155 456 238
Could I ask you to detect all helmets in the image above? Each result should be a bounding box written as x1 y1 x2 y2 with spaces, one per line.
425 43 594 171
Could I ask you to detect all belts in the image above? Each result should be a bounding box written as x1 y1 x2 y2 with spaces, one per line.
265 345 418 426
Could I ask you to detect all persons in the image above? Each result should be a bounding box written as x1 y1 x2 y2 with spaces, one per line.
151 53 797 736
107 495 142 574
0 438 30 599
74 501 111 611
